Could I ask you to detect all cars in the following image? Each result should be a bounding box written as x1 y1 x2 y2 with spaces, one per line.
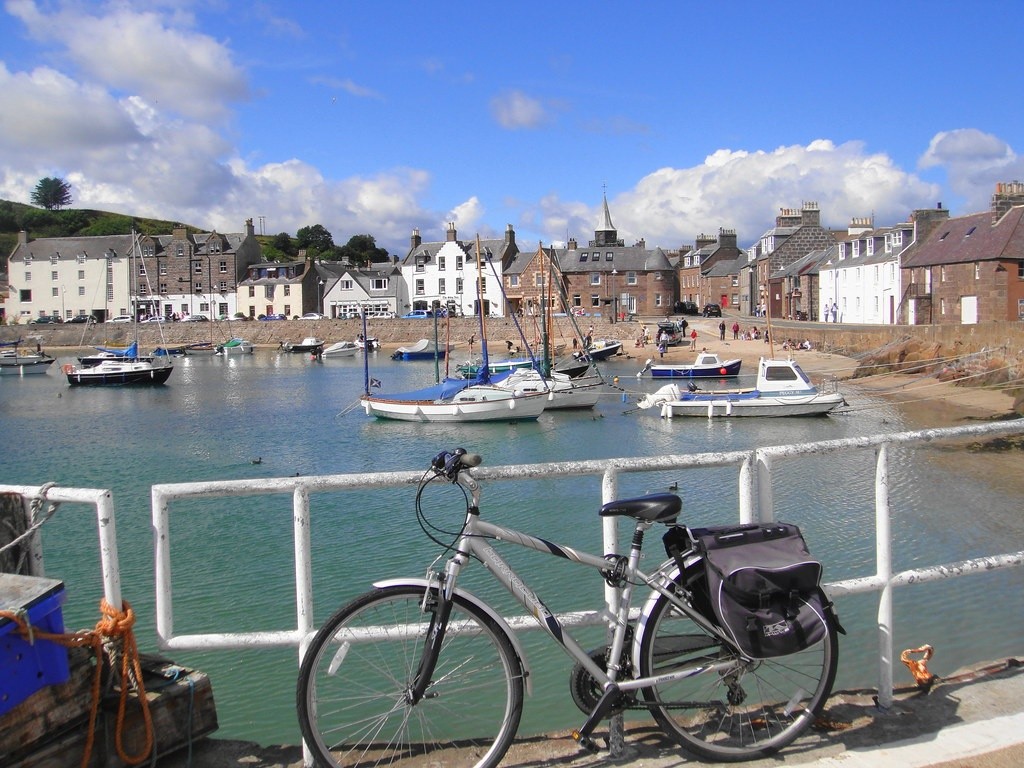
141 316 168 323
221 315 244 322
400 310 433 319
366 311 398 319
187 314 209 322
436 308 447 317
297 312 328 321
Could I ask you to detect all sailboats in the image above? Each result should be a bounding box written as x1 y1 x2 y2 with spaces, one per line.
152 294 253 357
75 258 155 365
359 233 623 424
62 226 175 386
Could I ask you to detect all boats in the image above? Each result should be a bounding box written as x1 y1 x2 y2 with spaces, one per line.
0 338 57 374
636 347 742 379
277 337 326 352
311 341 360 360
636 346 854 420
354 337 382 349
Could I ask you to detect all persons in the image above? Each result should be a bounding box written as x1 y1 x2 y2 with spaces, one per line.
823 303 838 323
573 326 595 349
467 332 476 344
741 326 768 343
676 317 686 337
782 338 811 350
732 321 739 339
689 329 697 351
172 312 185 321
506 341 513 349
571 306 584 316
138 313 153 322
360 334 364 341
702 348 707 353
755 303 765 317
719 321 725 340
635 323 650 348
658 331 669 357
89 318 96 327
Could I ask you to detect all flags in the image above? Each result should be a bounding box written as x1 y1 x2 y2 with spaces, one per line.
370 379 381 388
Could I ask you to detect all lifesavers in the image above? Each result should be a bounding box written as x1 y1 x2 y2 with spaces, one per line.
63 364 73 375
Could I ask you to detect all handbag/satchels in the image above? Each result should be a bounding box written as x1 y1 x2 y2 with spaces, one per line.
661 519 830 661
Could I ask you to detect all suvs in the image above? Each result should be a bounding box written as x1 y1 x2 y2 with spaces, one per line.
674 301 699 315
104 315 135 323
260 314 286 320
703 303 721 318
30 316 63 324
656 322 683 347
65 315 98 323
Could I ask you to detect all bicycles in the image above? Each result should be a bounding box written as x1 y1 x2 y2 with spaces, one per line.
294 447 849 768
